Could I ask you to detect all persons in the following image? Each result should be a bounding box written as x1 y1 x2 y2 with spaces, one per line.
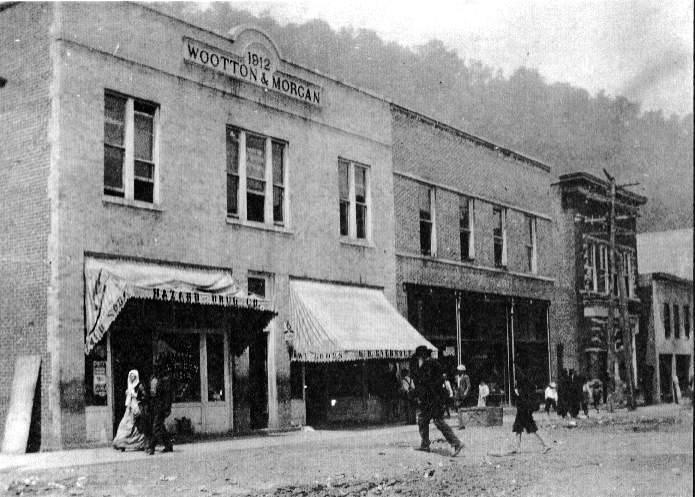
385 363 620 429
138 362 174 454
509 363 552 454
412 345 464 456
111 369 145 451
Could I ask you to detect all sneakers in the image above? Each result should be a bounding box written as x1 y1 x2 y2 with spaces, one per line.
414 446 430 453
450 439 465 456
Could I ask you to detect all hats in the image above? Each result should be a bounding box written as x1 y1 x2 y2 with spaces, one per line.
457 364 466 371
411 346 432 357
